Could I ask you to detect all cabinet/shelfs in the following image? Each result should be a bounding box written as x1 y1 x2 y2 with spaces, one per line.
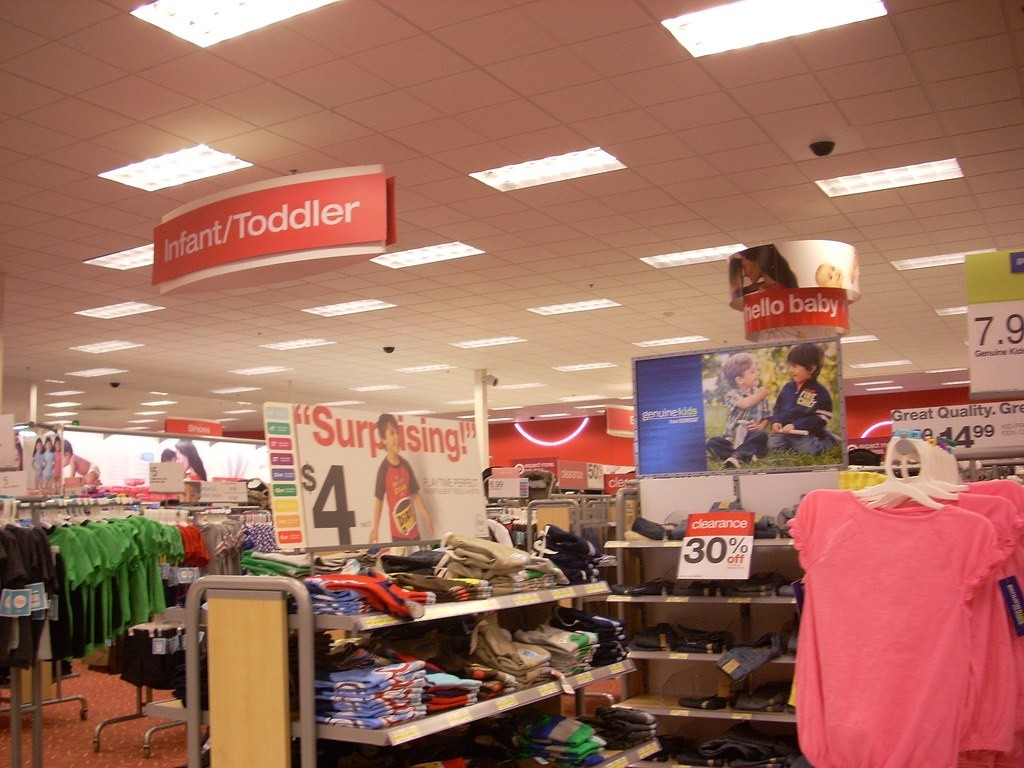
290 539 808 767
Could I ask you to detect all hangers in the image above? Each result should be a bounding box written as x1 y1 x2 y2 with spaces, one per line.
3 493 273 540
814 434 1024 536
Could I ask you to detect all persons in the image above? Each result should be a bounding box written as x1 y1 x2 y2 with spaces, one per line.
815 263 844 290
708 352 773 469
180 481 202 505
368 413 435 544
175 438 208 483
763 343 843 456
725 244 799 302
62 440 103 486
14 435 24 471
159 448 178 463
28 436 61 497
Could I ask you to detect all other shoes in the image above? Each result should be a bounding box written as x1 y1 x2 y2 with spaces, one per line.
723 457 741 469
